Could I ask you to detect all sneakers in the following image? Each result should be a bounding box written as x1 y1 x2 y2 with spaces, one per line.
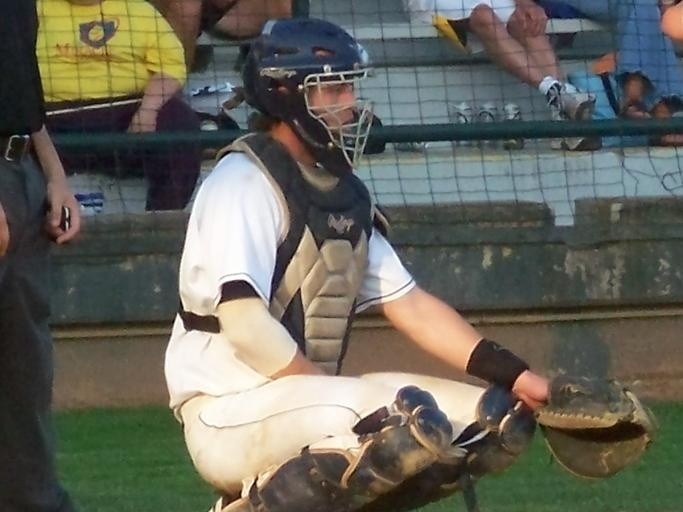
547 83 598 118
551 135 605 152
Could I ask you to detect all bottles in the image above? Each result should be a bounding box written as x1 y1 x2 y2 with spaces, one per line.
454 103 474 146
478 102 499 147
70 169 104 217
502 102 524 150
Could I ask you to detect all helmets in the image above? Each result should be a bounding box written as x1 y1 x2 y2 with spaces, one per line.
242 19 375 181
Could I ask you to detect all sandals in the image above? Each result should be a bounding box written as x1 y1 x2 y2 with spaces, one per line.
647 133 683 149
620 87 649 119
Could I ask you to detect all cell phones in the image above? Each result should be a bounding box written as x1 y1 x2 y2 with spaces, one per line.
43 206 70 232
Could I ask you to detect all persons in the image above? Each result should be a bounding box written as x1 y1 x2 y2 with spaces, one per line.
162 14 655 512
402 1 603 149
562 0 683 147
658 0 682 42
33 0 203 211
1 1 83 511
153 1 310 82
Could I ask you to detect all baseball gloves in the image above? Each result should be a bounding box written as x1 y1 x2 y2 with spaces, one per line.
535 375 654 481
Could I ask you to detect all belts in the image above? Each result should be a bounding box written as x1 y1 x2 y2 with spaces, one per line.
1 133 37 162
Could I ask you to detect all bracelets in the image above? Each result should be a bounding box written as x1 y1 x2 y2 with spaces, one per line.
468 336 528 394
